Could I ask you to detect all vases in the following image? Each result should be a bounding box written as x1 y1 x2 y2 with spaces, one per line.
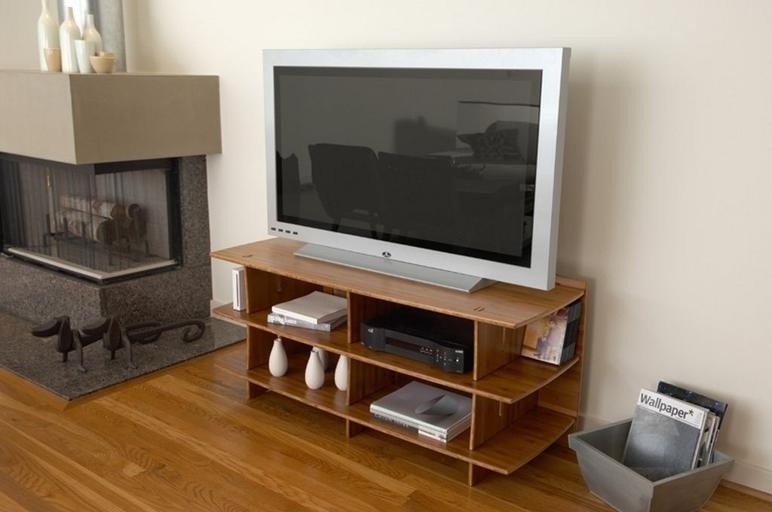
82 13 104 57
311 346 328 371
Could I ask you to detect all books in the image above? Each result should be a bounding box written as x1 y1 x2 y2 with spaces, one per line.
266 290 348 332
620 379 728 482
368 380 473 444
520 300 583 368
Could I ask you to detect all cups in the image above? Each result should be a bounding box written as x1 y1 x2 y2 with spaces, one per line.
76 39 95 74
96 51 113 56
43 48 60 71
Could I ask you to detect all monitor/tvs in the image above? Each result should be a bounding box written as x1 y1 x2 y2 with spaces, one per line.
263 47 572 292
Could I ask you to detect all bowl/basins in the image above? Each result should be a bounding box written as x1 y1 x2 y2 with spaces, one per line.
89 56 116 73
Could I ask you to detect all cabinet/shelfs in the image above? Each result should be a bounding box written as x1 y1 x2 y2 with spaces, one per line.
208 235 589 487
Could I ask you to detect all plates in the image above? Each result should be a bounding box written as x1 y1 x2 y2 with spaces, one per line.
449 159 485 169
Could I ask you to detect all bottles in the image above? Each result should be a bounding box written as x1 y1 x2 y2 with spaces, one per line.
82 15 102 53
312 346 328 369
